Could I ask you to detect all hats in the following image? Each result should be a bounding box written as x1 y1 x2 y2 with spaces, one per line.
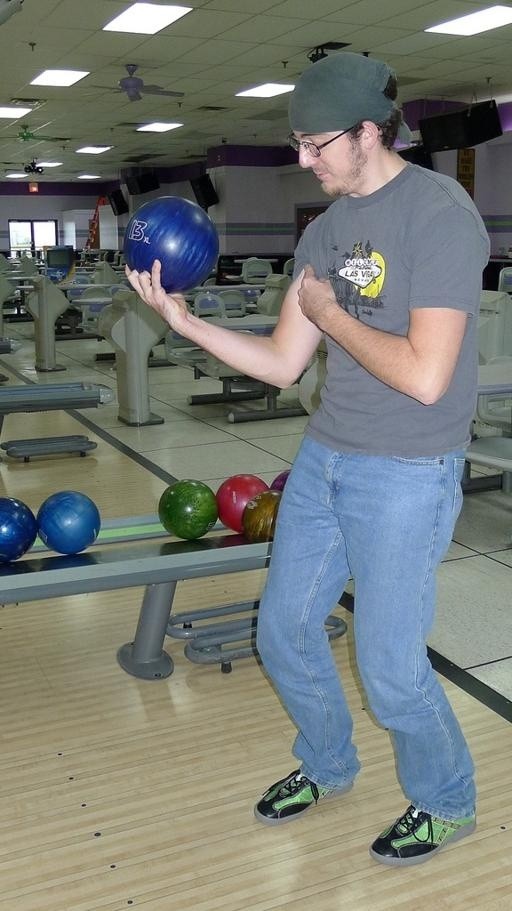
287 52 400 131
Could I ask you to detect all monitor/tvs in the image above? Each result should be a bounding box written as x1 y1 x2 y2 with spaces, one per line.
47 250 71 268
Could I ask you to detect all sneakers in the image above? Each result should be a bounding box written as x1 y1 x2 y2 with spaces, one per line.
254 768 354 827
369 803 476 867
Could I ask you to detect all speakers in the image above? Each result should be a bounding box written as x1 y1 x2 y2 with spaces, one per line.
418 100 503 155
127 171 160 195
108 190 129 216
190 174 218 208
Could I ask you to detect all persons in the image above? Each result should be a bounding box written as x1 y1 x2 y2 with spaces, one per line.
123 48 492 865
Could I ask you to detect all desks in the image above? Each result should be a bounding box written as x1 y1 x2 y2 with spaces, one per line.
0 262 124 294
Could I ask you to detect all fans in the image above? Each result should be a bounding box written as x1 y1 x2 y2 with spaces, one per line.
89 65 184 101
0 124 57 142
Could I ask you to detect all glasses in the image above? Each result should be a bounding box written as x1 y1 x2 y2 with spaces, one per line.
285 122 362 157
